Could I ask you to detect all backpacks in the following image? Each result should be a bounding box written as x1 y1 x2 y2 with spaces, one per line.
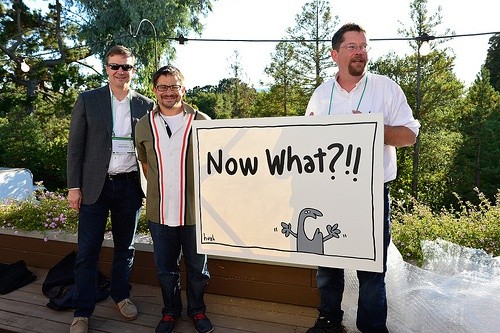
0 259 37 295
41 248 111 311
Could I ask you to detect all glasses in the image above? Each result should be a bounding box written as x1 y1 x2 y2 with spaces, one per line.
339 42 372 53
154 85 181 91
106 63 134 71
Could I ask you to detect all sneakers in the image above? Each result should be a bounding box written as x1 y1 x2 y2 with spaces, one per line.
190 312 215 333
155 313 182 333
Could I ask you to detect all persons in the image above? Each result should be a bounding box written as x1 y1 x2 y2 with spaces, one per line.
134 65 215 333
305 24 421 333
67 46 155 333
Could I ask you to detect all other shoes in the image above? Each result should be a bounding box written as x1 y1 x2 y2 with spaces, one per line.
357 325 389 333
70 316 89 333
112 298 138 320
305 310 347 333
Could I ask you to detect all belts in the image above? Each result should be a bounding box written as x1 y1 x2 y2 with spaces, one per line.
106 172 138 180
